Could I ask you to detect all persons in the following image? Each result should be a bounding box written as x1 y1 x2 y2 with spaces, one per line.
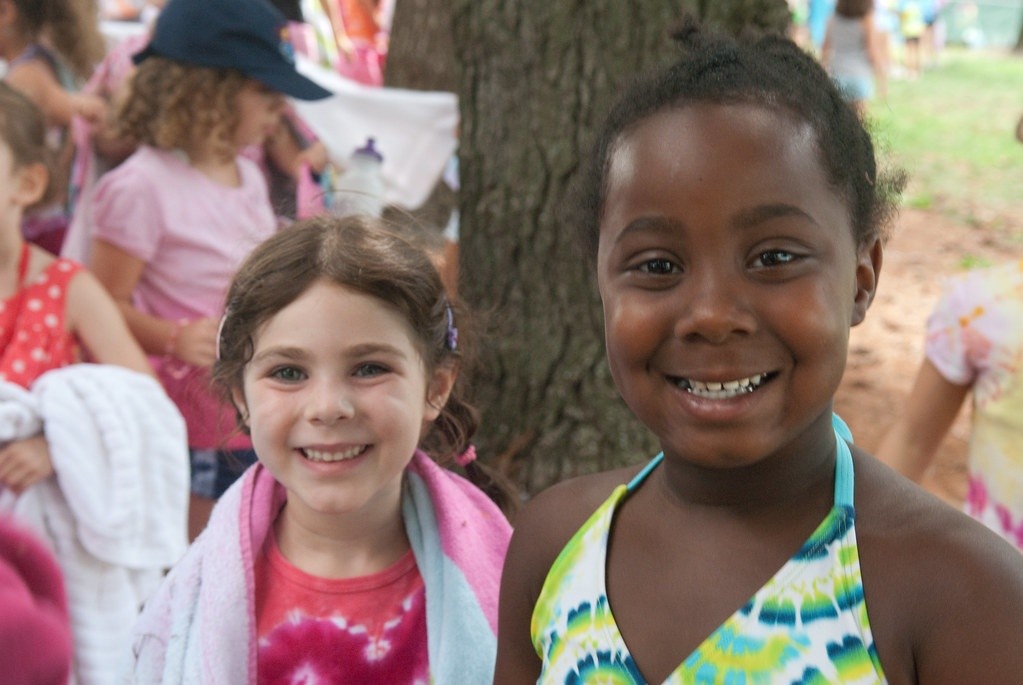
872 114 1023 551
0 1 460 684
491 33 1023 685
808 1 1022 120
131 189 514 685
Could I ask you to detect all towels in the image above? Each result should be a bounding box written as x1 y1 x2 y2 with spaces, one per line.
133 446 514 684
1 362 190 685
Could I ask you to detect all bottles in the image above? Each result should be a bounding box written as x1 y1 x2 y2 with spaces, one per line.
338 138 384 211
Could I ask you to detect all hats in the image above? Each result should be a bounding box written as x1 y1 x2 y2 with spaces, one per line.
134 0 332 100
